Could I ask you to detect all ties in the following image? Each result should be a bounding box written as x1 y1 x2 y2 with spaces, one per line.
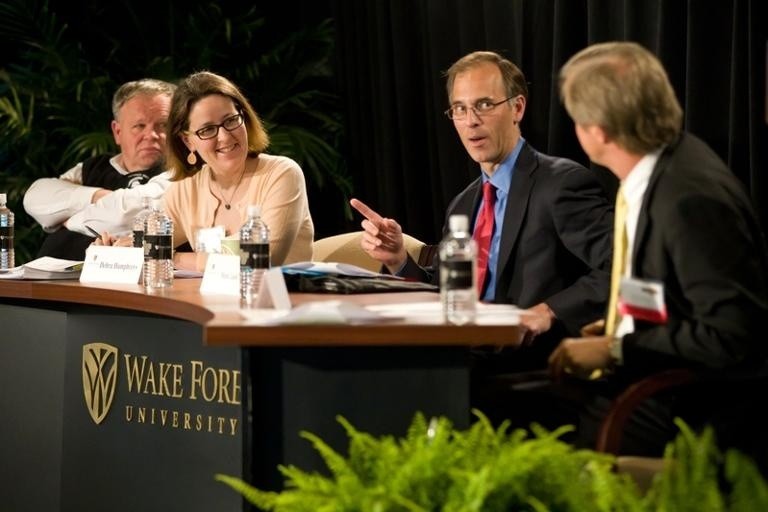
589 186 628 380
473 182 498 300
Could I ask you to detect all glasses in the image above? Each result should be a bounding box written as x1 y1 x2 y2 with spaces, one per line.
444 96 514 120
180 110 245 140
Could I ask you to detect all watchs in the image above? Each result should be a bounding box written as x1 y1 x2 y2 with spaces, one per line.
606 337 623 365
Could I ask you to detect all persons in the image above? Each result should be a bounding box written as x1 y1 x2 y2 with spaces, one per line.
546 40 766 459
348 50 615 376
89 69 315 272
21 77 179 260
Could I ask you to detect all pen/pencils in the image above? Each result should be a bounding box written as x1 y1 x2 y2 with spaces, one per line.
84 225 102 239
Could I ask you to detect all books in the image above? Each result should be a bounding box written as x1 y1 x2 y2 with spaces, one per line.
1 256 83 280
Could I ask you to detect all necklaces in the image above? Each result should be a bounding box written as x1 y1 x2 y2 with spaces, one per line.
216 173 243 208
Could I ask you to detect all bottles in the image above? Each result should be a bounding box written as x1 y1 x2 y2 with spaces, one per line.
438 214 479 326
0 193 15 274
238 206 270 311
131 195 174 290
